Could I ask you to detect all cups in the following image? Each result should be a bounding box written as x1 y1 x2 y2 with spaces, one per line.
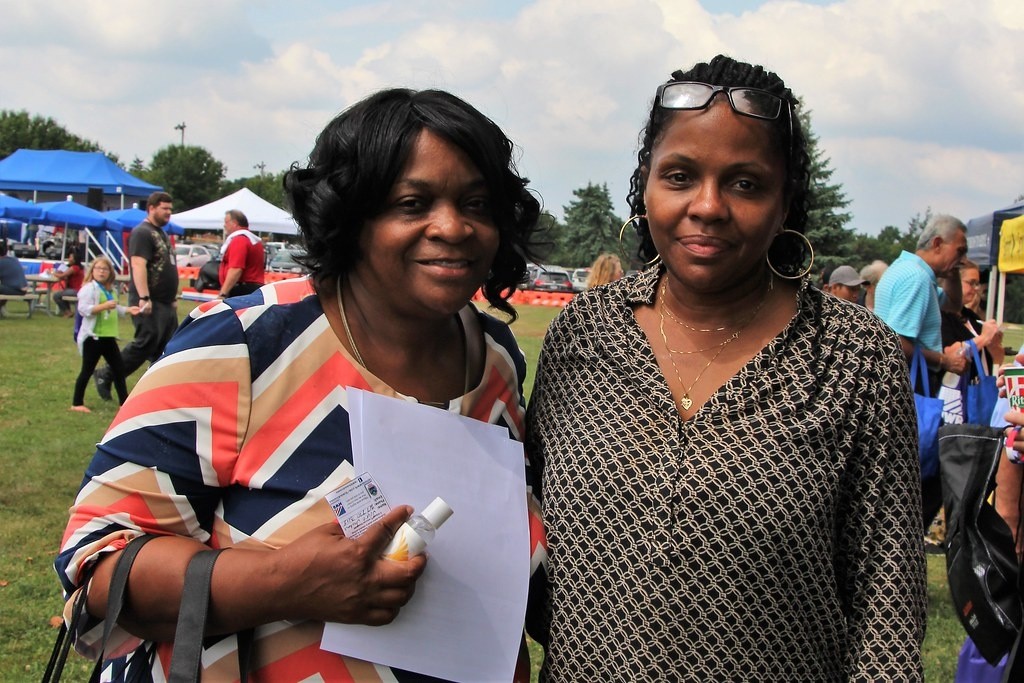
1001 367 1024 462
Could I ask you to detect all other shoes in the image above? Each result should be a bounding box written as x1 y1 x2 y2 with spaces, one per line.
925 531 945 551
70 406 90 413
63 311 74 318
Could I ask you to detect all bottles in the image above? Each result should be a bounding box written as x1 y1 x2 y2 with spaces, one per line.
941 343 971 388
378 495 454 563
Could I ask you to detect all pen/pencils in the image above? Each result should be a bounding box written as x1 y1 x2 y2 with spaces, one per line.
976 319 983 324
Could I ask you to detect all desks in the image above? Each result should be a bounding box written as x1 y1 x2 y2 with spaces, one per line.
24 273 61 318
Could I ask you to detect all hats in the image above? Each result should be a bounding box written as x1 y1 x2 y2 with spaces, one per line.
829 266 870 287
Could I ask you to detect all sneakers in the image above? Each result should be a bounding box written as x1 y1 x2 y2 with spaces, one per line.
94 369 113 401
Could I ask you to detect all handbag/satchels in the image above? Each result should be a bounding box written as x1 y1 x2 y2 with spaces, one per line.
965 338 999 426
910 344 944 478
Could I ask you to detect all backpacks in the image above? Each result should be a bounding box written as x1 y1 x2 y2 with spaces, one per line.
938 424 1024 668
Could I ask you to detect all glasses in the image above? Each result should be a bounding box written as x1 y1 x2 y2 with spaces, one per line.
647 81 793 160
94 266 109 271
963 280 980 286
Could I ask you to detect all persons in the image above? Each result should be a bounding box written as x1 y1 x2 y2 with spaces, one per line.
92 191 179 403
217 210 264 299
527 56 926 683
23 223 38 246
53 253 85 319
0 241 27 319
55 90 554 683
78 229 89 260
822 214 1003 554
67 256 140 413
991 342 1024 683
50 226 65 239
586 253 624 289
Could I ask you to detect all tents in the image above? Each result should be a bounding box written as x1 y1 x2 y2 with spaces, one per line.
169 187 303 274
0 148 164 210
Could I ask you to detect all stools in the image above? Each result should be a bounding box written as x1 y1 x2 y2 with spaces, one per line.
0 293 39 319
21 288 53 307
62 295 79 314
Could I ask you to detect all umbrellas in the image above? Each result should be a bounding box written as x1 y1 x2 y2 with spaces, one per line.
0 195 184 261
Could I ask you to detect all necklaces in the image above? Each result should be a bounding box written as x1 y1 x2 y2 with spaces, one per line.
947 306 968 323
337 271 471 405
658 271 774 410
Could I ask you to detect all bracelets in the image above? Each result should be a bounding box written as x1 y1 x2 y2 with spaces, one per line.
931 351 944 372
219 294 226 297
140 296 151 301
1006 425 1024 465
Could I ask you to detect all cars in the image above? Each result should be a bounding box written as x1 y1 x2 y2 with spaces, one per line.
173 240 309 275
518 262 640 293
